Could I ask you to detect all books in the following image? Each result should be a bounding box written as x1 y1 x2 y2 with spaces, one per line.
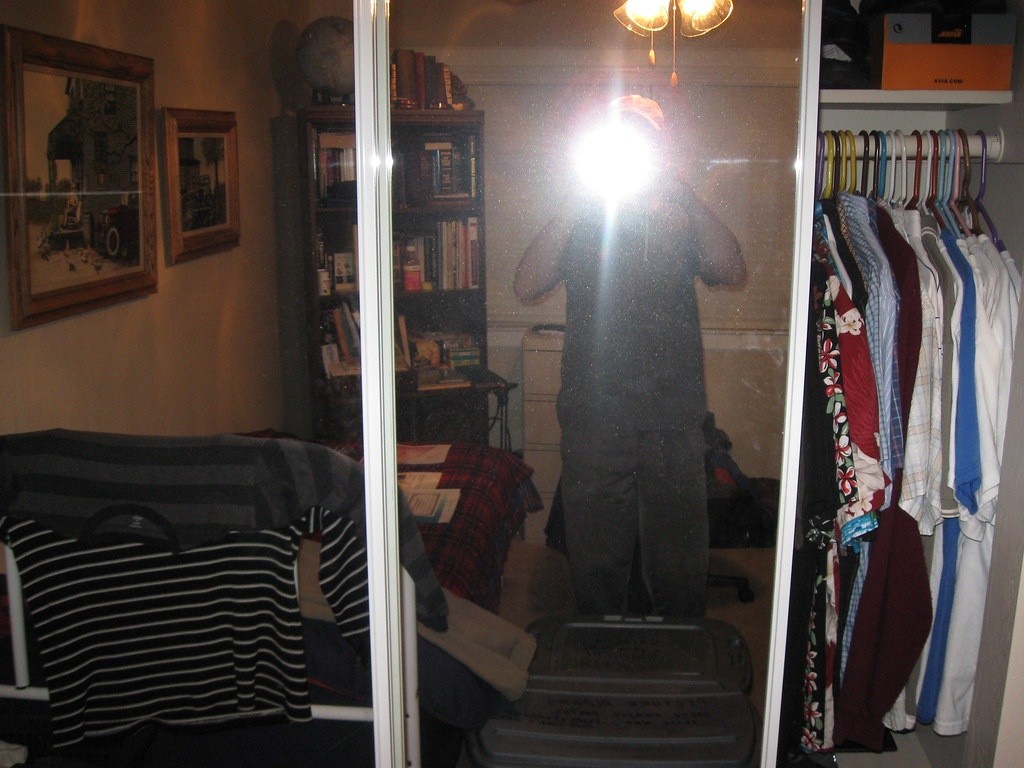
390 49 453 110
317 137 479 391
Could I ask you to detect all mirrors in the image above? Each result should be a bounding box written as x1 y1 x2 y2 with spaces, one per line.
0 0 825 768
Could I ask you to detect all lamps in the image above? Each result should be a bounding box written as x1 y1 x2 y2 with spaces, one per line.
613 0 734 87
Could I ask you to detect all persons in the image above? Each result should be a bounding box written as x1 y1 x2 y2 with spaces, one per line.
63 184 78 227
513 97 747 616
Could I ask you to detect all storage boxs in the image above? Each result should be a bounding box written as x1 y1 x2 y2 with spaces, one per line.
882 12 1017 91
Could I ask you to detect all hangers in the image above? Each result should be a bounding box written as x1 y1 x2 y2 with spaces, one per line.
815 129 1006 252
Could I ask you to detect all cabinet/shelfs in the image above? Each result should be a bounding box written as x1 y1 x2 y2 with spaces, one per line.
295 104 489 444
521 326 564 541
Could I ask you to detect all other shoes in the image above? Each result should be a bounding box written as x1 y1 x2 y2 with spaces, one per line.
819 0 875 89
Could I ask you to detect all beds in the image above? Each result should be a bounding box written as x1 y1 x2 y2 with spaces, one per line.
0 428 546 768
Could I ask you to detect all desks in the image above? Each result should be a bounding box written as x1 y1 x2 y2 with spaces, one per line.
325 365 519 450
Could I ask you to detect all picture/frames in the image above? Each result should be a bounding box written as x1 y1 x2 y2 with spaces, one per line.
157 106 243 268
0 23 158 331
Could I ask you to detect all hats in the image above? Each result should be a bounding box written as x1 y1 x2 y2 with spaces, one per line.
603 96 664 131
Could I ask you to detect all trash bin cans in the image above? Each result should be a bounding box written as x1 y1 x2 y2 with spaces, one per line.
466 607 764 768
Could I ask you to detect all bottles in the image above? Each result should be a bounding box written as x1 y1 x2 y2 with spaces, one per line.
404 247 423 291
